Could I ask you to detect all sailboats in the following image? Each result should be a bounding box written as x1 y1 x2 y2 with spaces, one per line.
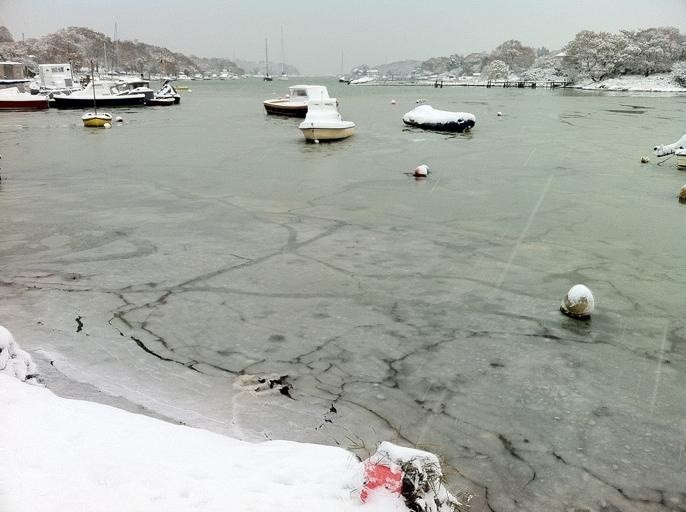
279 24 289 81
264 36 272 81
81 59 112 127
338 46 348 83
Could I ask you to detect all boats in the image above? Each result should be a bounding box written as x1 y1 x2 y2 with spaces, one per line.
0 83 49 108
298 91 355 142
672 133 686 171
53 80 150 110
402 105 476 131
263 85 339 119
156 79 181 104
140 69 249 82
173 84 188 91
146 98 175 107
